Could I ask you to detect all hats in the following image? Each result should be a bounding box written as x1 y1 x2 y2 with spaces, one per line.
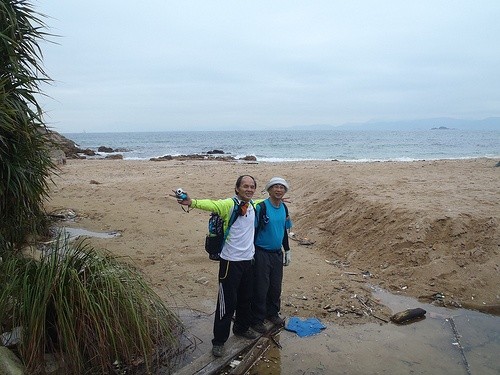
266 178 289 194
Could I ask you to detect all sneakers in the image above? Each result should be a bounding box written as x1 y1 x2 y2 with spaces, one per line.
253 321 267 332
269 314 283 325
212 344 223 357
241 330 256 339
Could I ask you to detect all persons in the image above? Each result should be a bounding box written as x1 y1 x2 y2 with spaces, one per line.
169 175 259 357
251 177 292 332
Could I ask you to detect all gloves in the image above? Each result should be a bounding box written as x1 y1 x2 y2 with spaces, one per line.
284 250 291 266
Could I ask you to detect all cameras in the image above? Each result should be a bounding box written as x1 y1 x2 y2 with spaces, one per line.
176 188 188 201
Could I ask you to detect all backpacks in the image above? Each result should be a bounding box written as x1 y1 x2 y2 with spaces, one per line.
205 198 255 261
